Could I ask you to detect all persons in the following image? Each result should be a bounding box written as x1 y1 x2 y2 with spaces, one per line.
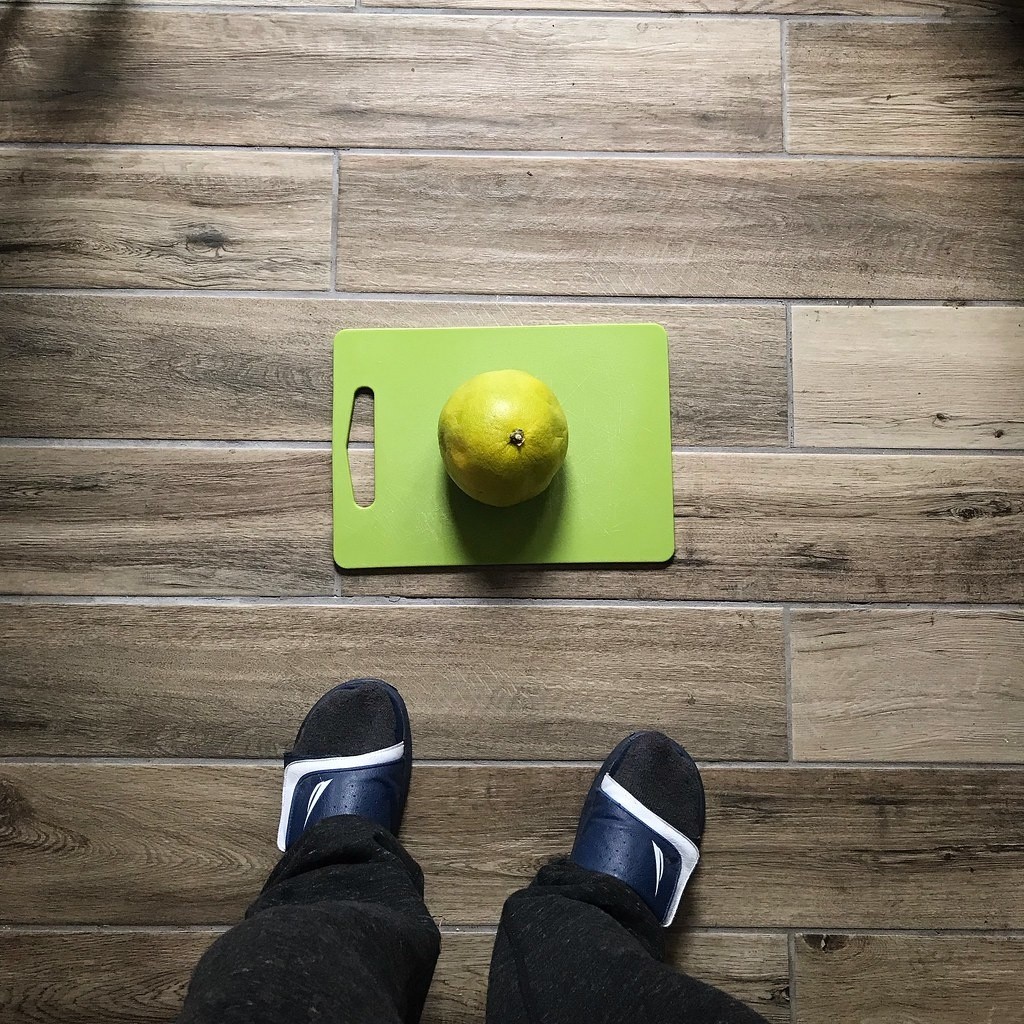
175 675 774 1024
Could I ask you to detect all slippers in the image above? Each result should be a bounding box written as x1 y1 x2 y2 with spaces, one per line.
278 676 413 857
566 728 705 931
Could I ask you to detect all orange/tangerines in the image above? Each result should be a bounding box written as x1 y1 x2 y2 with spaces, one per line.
437 370 569 507
